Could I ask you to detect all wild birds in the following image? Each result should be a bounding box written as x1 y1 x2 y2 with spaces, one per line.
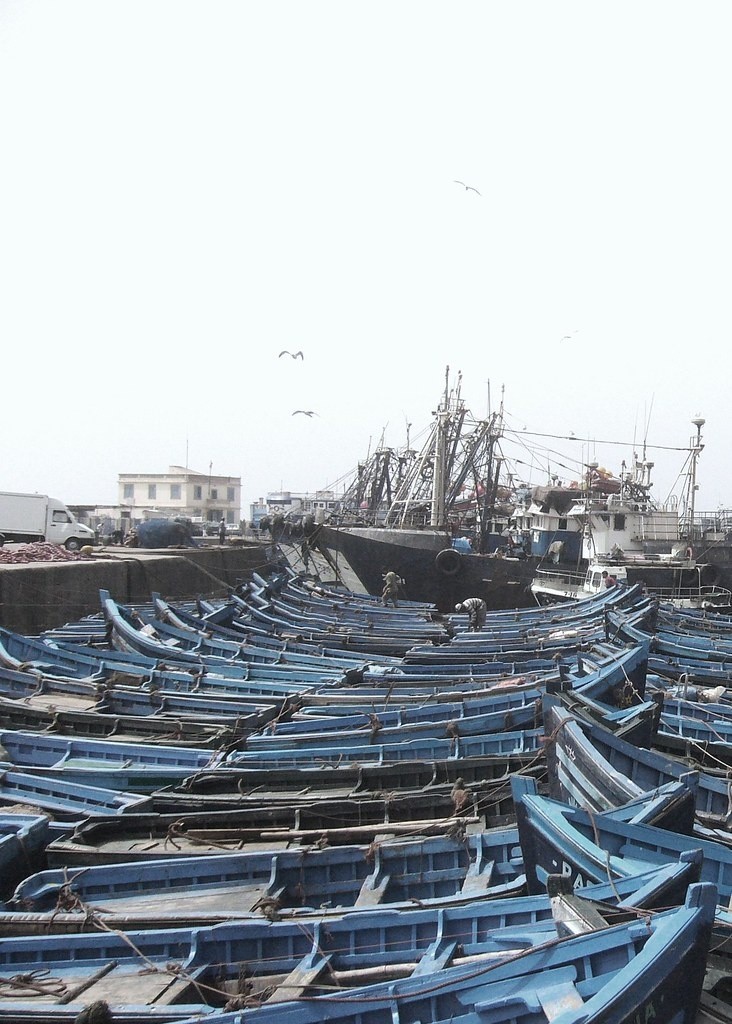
453 180 481 195
291 411 319 417
279 351 303 360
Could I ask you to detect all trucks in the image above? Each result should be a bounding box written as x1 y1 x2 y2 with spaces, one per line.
0 491 95 551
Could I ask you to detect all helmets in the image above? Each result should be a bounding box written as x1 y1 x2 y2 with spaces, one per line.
454 603 462 613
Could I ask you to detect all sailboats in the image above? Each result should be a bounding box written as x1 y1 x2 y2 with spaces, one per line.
259 364 732 616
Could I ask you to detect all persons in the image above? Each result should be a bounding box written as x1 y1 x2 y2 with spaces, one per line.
240 519 246 535
602 571 618 587
549 539 568 565
219 517 226 545
249 517 259 540
455 598 486 632
97 522 104 531
103 527 125 546
381 566 398 608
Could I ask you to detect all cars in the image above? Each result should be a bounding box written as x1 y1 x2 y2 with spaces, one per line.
224 524 239 535
207 523 219 536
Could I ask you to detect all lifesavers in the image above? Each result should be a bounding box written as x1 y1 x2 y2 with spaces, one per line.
274 505 279 511
436 548 462 575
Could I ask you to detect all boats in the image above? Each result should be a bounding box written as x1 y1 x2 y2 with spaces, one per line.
0 568 732 1024
530 543 732 613
